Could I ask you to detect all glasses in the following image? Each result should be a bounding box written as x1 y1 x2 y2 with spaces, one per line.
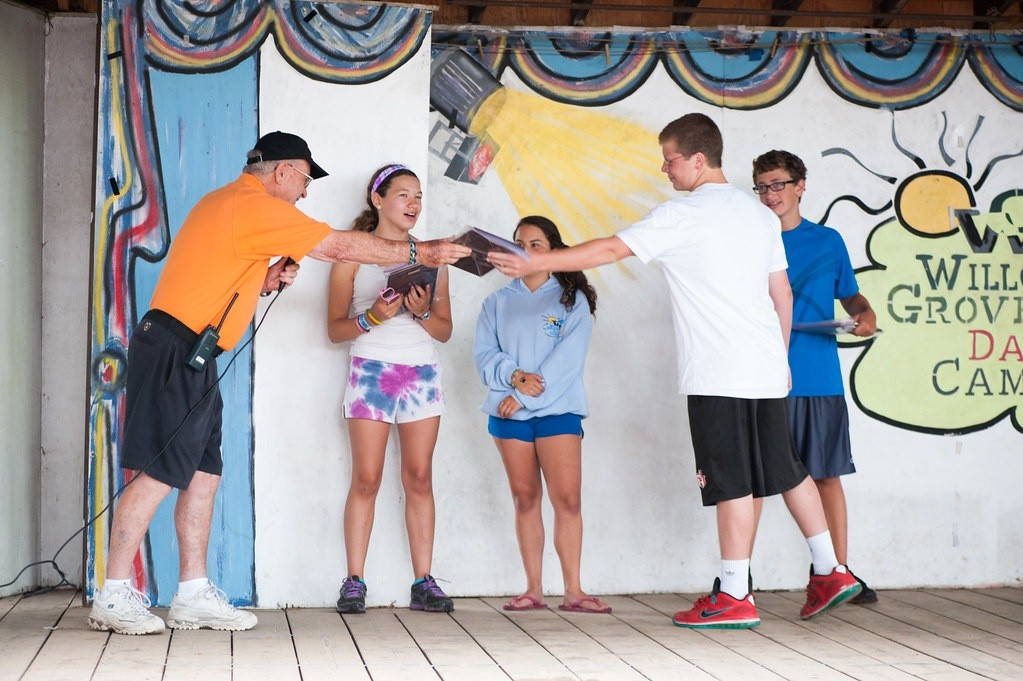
664 150 705 165
752 180 796 194
274 161 313 188
381 286 400 305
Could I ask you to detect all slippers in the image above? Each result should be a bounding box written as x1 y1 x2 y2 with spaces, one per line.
557 598 612 613
503 595 548 610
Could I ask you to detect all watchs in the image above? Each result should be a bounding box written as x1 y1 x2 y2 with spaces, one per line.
412 305 431 321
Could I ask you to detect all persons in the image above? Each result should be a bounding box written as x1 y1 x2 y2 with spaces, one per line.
87 131 472 634
473 215 610 615
327 163 453 613
486 114 861 628
746 149 876 606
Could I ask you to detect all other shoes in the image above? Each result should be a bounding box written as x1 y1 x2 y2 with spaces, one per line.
844 564 878 605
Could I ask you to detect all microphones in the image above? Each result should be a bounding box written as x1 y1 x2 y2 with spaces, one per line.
278 257 294 292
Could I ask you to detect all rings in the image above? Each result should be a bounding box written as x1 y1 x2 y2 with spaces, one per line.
500 402 503 406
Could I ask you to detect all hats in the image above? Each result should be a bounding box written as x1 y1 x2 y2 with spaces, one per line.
247 131 330 179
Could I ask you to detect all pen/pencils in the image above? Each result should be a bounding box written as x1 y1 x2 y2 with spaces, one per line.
413 314 415 321
854 322 860 327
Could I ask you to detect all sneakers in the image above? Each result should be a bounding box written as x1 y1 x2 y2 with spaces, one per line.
166 580 258 631
672 577 761 629
337 574 367 613
86 584 165 635
408 572 455 612
799 563 863 621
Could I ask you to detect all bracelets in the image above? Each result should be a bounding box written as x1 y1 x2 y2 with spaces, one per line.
355 309 382 333
407 239 417 265
510 369 523 387
259 292 271 297
